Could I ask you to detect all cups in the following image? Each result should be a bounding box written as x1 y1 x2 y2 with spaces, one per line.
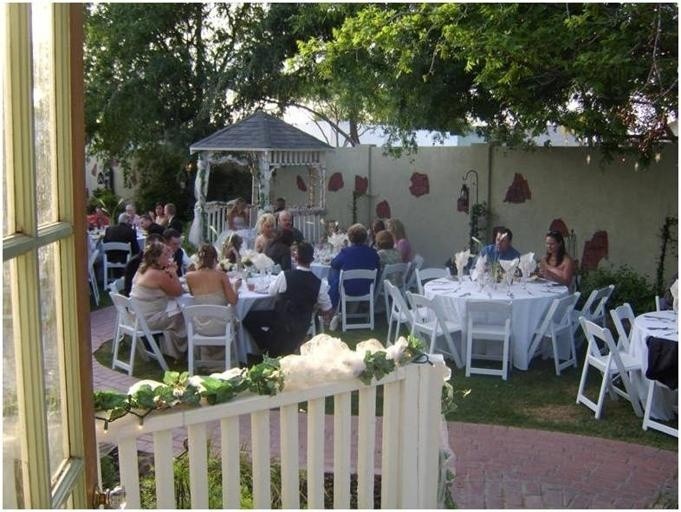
447 266 532 294
247 278 258 291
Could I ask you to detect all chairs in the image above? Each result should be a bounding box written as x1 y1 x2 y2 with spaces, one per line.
407 252 422 287
105 292 172 377
458 296 517 381
401 289 461 372
573 316 646 419
378 262 409 319
564 282 615 359
608 302 639 354
522 291 580 378
89 230 149 308
180 301 242 377
637 329 678 436
108 276 149 361
652 295 671 309
334 267 379 333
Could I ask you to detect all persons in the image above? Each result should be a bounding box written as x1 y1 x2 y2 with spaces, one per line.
88 198 413 368
537 231 574 297
470 226 523 277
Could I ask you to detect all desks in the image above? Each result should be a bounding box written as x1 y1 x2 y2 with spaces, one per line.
621 308 678 425
288 240 379 291
165 268 289 361
415 270 574 371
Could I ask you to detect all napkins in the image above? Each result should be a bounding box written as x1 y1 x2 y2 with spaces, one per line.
518 250 536 276
471 254 492 272
454 249 472 266
499 258 519 277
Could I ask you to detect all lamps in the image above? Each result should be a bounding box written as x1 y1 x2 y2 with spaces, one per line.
458 168 478 204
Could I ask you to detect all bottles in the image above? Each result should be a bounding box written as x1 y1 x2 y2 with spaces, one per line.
259 267 273 291
312 238 333 265
290 242 299 263
